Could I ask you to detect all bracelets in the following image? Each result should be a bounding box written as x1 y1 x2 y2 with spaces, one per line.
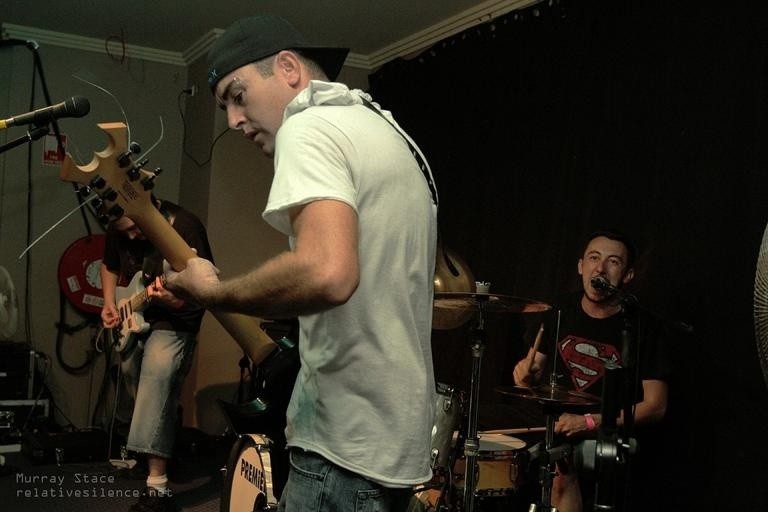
582 411 597 432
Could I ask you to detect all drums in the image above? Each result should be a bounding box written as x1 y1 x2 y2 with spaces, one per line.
449 430 528 497
430 381 460 469
220 434 289 511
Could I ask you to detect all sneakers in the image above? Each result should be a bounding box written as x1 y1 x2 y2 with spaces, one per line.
128 485 181 512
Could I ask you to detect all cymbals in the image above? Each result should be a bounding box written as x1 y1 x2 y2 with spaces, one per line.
432 292 553 313
496 385 600 405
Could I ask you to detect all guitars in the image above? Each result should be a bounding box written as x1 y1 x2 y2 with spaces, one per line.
59 122 300 430
109 269 179 353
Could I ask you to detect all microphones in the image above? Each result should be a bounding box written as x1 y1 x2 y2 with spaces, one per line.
590 274 609 291
0 95 91 130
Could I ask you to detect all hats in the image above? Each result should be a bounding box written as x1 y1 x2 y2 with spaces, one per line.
205 14 351 96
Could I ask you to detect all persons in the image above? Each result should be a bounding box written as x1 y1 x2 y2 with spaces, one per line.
98 193 216 511
509 226 671 511
159 10 446 510
